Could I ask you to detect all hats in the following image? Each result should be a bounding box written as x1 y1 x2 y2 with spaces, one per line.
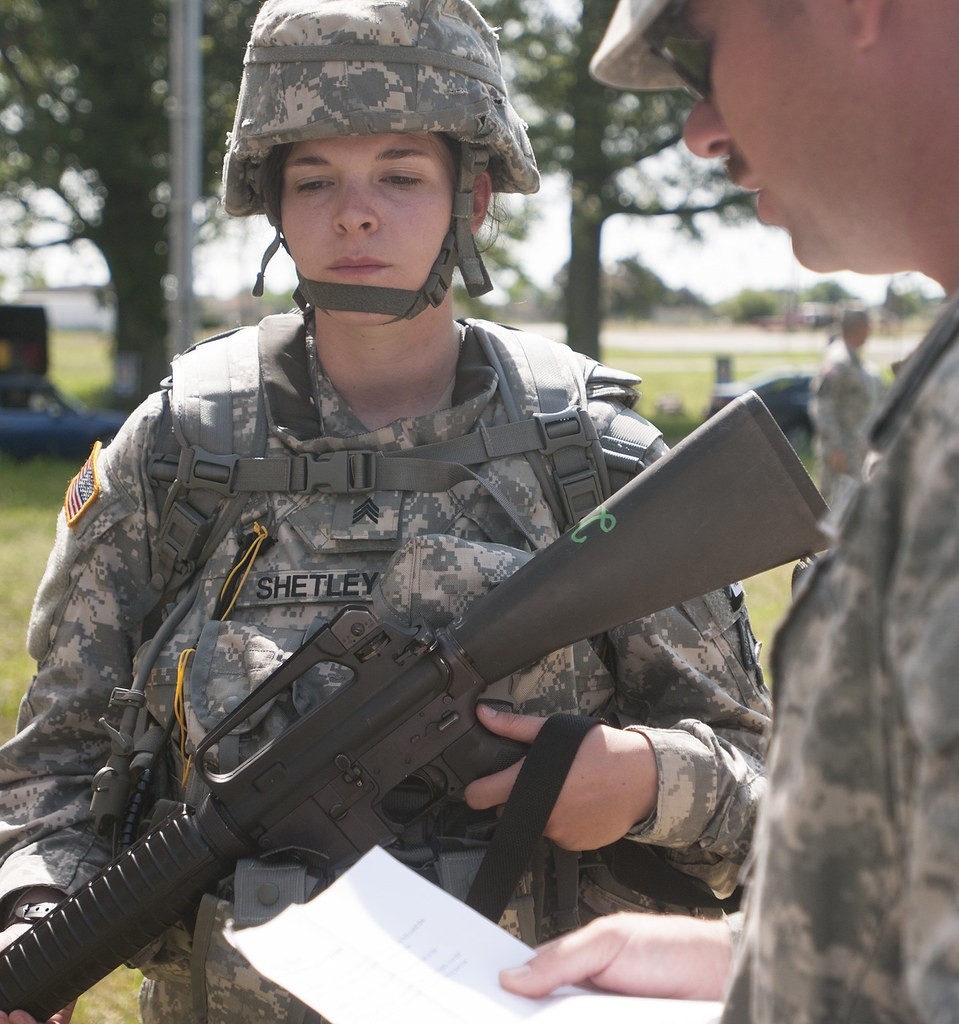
583 1 713 108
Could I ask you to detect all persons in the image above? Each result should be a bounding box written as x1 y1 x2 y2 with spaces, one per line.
500 0 956 1022
0 4 770 1022
809 307 873 517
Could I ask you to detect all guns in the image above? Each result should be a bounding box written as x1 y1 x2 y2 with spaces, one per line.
0 383 839 1021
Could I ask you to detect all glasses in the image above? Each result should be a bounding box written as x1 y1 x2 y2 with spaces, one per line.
645 12 714 112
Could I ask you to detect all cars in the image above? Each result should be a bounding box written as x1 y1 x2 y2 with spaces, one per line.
707 373 823 449
0 373 130 454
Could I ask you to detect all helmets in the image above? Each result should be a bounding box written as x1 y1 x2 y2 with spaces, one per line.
218 0 540 230
